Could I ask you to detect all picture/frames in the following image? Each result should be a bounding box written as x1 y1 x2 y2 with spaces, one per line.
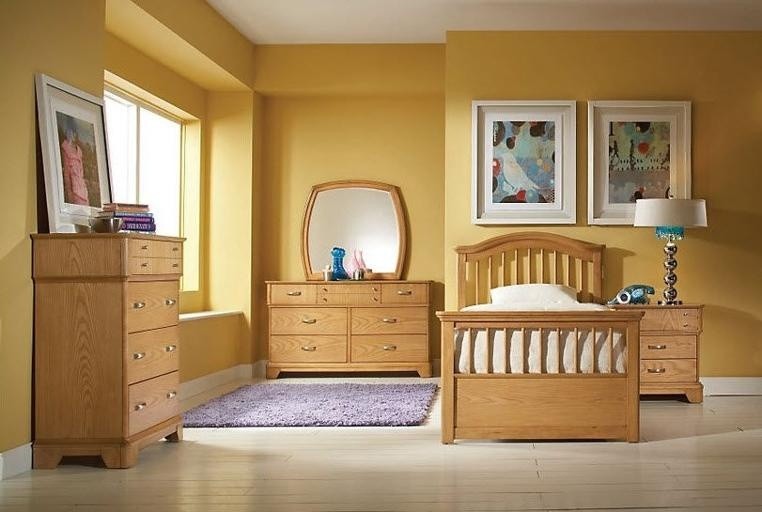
35 73 114 234
471 100 576 225
587 100 692 226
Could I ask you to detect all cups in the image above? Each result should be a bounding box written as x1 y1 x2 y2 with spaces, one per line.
354 268 364 281
89 218 125 233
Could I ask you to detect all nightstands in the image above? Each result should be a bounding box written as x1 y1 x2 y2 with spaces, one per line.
615 305 704 402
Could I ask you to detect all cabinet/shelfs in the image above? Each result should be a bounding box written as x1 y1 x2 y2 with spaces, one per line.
263 281 434 379
31 234 182 468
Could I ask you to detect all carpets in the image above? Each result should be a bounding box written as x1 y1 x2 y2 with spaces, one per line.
179 383 437 427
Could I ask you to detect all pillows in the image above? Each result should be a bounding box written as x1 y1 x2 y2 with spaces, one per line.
489 283 579 306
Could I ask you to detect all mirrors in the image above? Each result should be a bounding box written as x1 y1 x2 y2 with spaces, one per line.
300 180 407 281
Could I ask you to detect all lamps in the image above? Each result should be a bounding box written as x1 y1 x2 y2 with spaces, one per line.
634 199 709 305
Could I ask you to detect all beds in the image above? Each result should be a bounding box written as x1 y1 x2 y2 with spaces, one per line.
434 231 646 443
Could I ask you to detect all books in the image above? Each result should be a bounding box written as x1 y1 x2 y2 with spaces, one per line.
96 202 156 236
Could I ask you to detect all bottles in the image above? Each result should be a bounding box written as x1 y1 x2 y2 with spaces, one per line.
323 265 332 280
